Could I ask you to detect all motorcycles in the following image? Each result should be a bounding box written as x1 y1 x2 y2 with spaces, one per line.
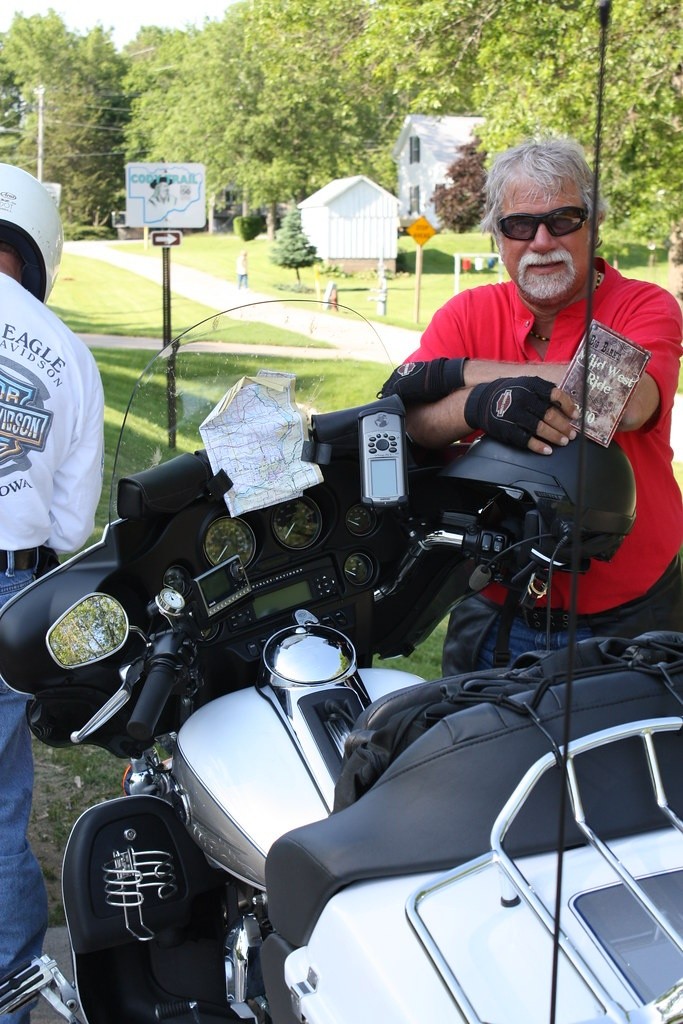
0 300 683 1024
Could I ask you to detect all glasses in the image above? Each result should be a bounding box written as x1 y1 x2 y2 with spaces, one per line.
499 205 589 242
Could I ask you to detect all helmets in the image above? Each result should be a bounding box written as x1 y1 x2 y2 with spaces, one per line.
0 163 63 303
441 420 637 561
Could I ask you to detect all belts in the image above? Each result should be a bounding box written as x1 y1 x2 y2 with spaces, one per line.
518 554 681 634
0 545 44 571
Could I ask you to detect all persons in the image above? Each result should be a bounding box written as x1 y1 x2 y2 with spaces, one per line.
388 134 678 678
0 164 111 1019
